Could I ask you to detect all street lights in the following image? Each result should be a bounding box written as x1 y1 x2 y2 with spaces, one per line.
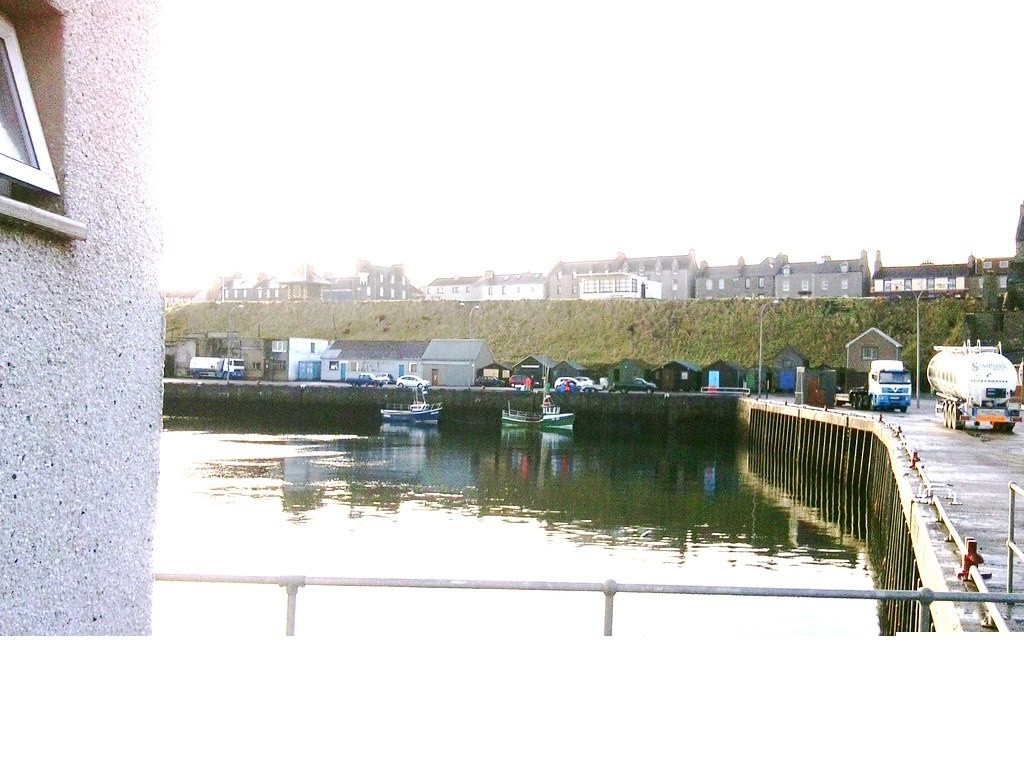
758 300 779 399
227 305 244 384
468 306 480 388
887 280 952 410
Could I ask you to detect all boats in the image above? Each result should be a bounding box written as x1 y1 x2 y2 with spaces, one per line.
502 358 576 430
381 389 443 424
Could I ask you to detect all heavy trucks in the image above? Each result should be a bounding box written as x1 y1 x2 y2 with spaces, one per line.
835 362 912 414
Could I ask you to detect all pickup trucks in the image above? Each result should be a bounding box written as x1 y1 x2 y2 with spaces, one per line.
345 374 387 387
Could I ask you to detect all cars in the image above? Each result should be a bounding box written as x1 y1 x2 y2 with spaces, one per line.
509 375 540 388
555 376 591 390
396 375 432 389
613 378 657 395
475 376 505 387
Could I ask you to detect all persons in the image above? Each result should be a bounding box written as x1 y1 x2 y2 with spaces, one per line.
526 377 531 391
530 375 535 391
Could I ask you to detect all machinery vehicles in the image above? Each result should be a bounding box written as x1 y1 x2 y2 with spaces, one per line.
187 357 246 380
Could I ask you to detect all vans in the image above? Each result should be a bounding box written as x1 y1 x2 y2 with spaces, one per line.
374 373 394 384
